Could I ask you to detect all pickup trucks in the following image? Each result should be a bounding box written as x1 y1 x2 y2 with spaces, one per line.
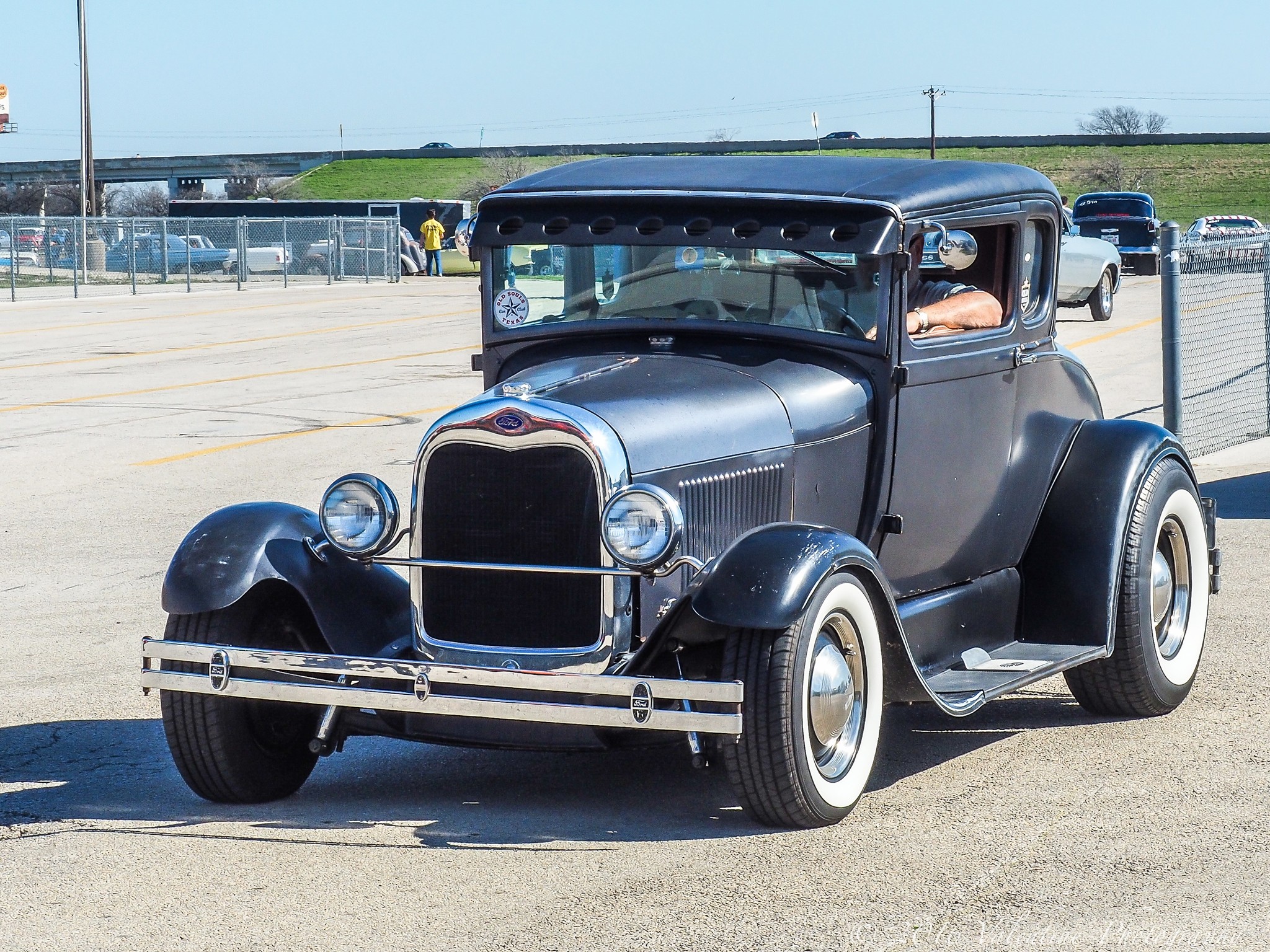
179 235 289 274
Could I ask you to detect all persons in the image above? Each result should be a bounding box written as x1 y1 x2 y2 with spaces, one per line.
1061 196 1073 217
778 234 1002 340
42 227 82 271
419 209 445 277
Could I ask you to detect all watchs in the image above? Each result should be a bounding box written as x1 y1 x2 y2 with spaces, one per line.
911 306 929 333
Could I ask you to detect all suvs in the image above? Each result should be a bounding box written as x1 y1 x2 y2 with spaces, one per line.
139 153 1221 829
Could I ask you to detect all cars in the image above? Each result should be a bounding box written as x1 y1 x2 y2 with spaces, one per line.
108 234 231 274
0 250 42 269
419 142 456 149
922 207 1123 321
1070 191 1161 275
290 223 428 276
1184 215 1270 274
0 230 11 251
15 228 44 252
168 198 472 276
817 131 861 138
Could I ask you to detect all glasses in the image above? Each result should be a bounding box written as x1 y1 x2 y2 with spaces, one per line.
855 237 919 261
